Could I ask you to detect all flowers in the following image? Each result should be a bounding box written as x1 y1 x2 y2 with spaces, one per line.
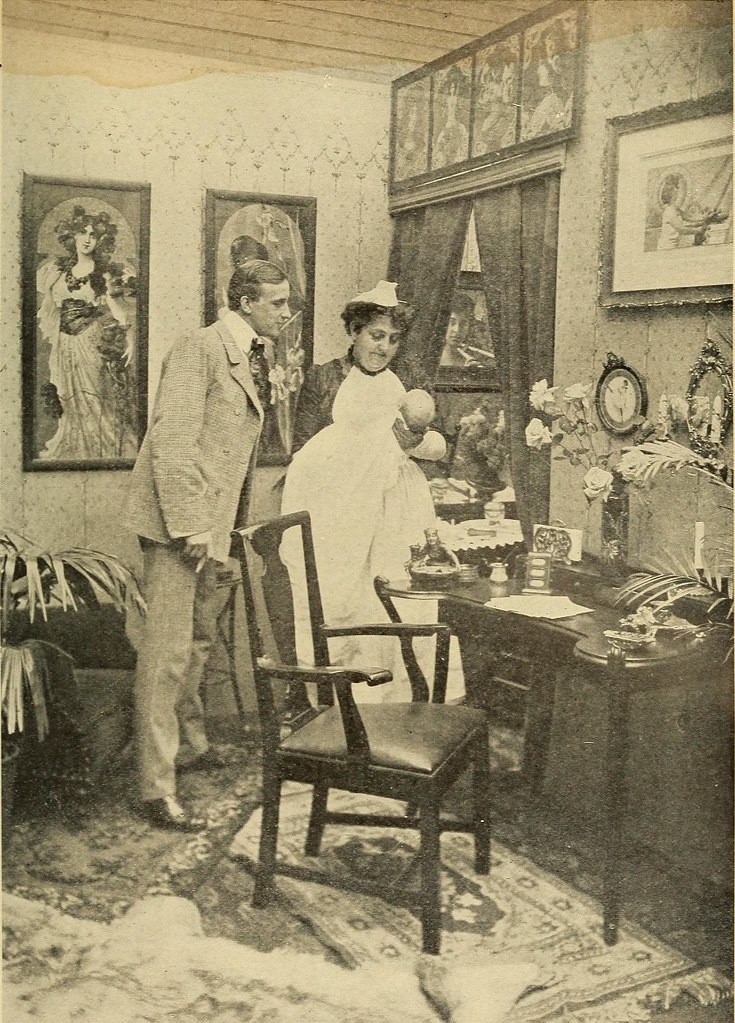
525 379 615 503
616 437 724 488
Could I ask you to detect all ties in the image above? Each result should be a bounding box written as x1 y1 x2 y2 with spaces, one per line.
250 338 272 454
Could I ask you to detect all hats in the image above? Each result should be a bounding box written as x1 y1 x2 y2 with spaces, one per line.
350 279 399 307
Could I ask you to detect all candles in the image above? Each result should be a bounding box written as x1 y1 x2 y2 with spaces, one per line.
695 522 705 569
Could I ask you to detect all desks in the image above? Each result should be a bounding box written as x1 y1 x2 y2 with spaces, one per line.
381 577 734 946
197 576 244 721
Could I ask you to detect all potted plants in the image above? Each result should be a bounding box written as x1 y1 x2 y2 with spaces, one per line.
1 528 149 852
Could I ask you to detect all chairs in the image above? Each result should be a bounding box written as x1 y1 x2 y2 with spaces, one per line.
230 510 491 955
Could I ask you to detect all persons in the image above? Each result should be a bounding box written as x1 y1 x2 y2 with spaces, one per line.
118 260 290 833
607 380 633 422
412 526 461 571
440 293 482 368
280 279 465 705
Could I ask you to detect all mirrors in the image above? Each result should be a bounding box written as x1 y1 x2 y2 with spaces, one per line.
684 338 733 458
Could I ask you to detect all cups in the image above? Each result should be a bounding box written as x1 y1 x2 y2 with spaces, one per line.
489 562 508 583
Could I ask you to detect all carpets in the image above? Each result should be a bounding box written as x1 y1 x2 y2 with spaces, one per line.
1 705 734 1022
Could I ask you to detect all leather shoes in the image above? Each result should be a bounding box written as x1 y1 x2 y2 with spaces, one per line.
130 794 208 834
176 746 246 774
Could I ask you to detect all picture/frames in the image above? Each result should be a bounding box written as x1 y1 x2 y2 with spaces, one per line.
22 172 152 470
389 0 587 191
432 280 501 393
203 188 318 465
597 87 733 309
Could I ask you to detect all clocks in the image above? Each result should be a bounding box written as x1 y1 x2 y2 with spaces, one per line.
595 351 648 439
533 519 583 566
